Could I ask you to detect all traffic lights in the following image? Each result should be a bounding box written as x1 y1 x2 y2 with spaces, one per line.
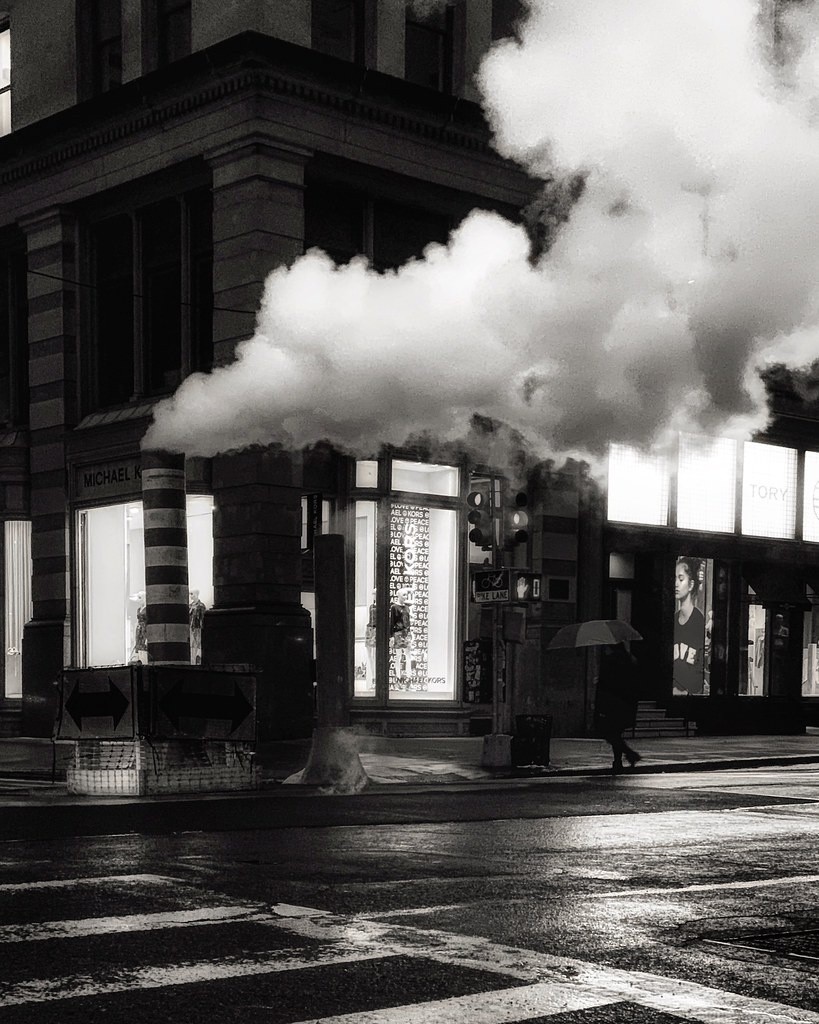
502 486 530 551
466 484 494 548
512 575 544 601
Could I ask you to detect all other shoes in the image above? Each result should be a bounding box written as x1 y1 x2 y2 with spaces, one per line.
632 757 641 767
608 761 624 773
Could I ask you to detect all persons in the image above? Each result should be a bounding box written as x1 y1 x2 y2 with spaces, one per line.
365 588 376 689
673 556 706 696
389 589 416 692
772 614 790 696
128 591 148 664
188 589 206 665
590 642 640 770
